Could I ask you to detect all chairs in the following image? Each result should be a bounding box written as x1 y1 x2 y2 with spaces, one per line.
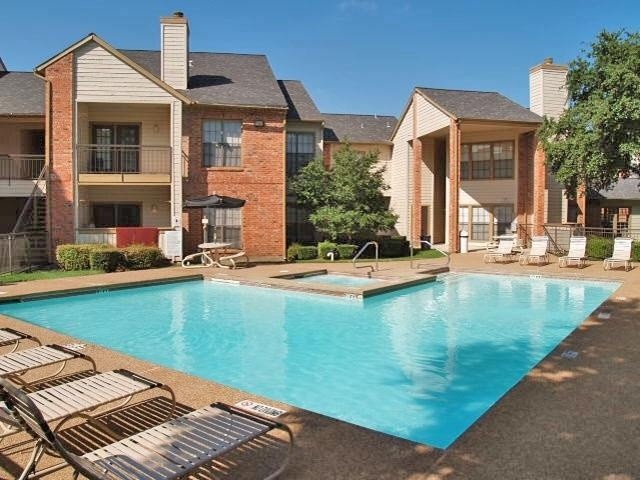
602 237 634 271
559 236 588 268
483 236 515 263
1 378 293 479
1 344 97 415
519 236 549 265
1 369 176 480
1 327 42 354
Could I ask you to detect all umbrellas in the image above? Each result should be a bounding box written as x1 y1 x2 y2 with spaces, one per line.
182 193 246 243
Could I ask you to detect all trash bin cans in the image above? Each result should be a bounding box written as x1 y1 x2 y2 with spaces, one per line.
422 236 430 250
460 231 468 253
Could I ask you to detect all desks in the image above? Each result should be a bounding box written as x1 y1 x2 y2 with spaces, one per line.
198 242 232 269
